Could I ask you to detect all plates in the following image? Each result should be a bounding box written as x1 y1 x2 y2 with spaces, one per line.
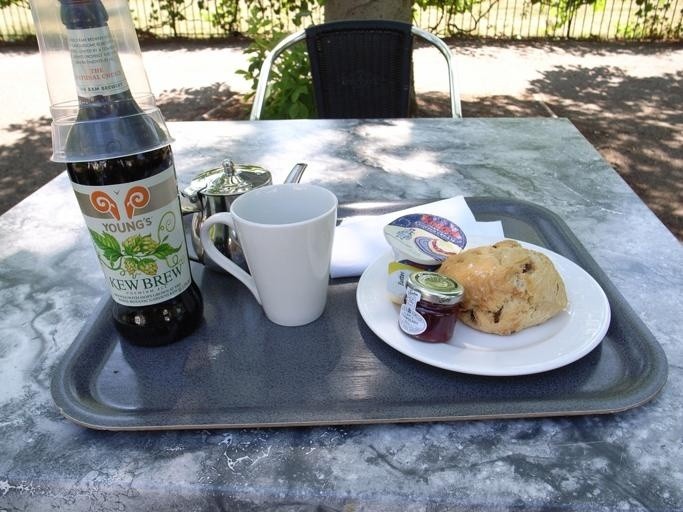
356 238 612 378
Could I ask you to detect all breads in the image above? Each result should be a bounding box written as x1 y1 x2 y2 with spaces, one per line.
437 239 567 335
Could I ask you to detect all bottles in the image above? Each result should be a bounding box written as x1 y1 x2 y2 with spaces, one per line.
59 0 204 350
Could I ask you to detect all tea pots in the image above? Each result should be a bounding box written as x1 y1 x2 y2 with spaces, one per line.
176 160 308 271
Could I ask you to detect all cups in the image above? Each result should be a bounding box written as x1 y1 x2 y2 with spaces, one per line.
28 0 178 164
199 182 338 326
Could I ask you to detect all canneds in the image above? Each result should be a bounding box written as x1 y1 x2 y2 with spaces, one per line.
399 271 465 343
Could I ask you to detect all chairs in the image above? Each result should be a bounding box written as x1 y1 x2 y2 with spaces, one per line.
249 20 462 121
